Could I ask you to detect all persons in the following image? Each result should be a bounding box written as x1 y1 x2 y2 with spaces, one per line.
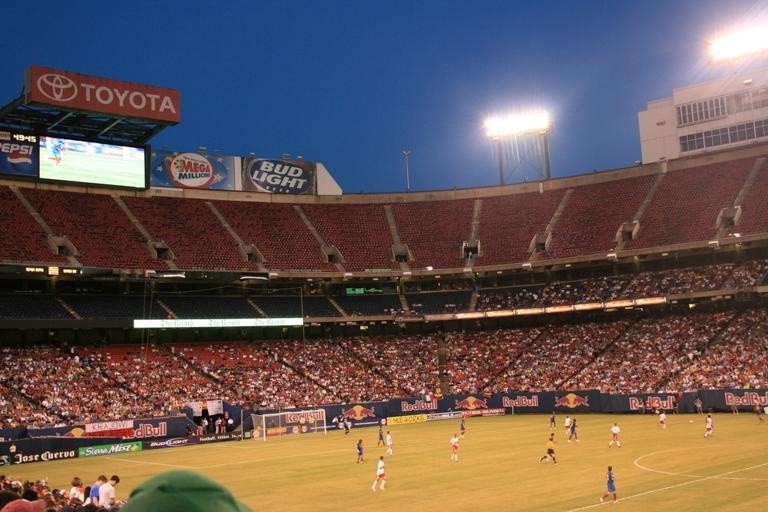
355 439 366 463
599 464 621 504
703 412 714 438
538 437 558 465
459 419 466 438
385 430 393 456
449 432 460 462
0 473 127 512
546 432 559 462
371 455 386 491
607 422 622 448
567 418 581 443
377 423 387 446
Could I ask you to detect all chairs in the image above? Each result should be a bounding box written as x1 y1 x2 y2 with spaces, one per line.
0 309 765 428
0 159 766 275
0 262 766 329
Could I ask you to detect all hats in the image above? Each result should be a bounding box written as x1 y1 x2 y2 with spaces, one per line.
0 497 47 512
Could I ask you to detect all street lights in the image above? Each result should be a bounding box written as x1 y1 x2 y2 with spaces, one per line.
400 145 412 192
